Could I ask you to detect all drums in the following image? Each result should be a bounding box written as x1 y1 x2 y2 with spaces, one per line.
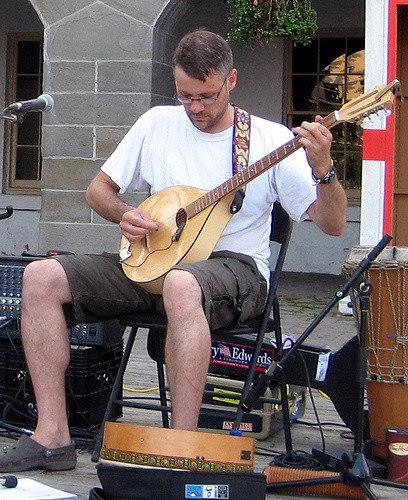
342 246 408 461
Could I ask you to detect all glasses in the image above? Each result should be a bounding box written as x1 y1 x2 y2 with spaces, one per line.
175 71 232 104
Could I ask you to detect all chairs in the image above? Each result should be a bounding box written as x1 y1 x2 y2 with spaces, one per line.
90 206 300 462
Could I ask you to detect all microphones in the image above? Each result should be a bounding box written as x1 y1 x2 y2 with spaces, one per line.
240 361 277 413
9 94 54 112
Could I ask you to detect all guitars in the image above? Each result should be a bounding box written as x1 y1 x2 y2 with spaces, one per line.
119 84 394 296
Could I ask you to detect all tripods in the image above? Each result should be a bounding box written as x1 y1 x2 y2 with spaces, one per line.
266 233 408 500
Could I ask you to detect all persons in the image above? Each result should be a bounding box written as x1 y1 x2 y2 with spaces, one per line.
0 29 347 474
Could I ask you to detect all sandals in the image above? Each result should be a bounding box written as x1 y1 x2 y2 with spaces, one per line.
0 433 78 472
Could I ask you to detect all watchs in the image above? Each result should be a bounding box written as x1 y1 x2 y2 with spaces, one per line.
311 159 336 186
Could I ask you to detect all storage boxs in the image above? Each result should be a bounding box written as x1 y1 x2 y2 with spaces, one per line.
197 373 307 440
0 340 125 431
